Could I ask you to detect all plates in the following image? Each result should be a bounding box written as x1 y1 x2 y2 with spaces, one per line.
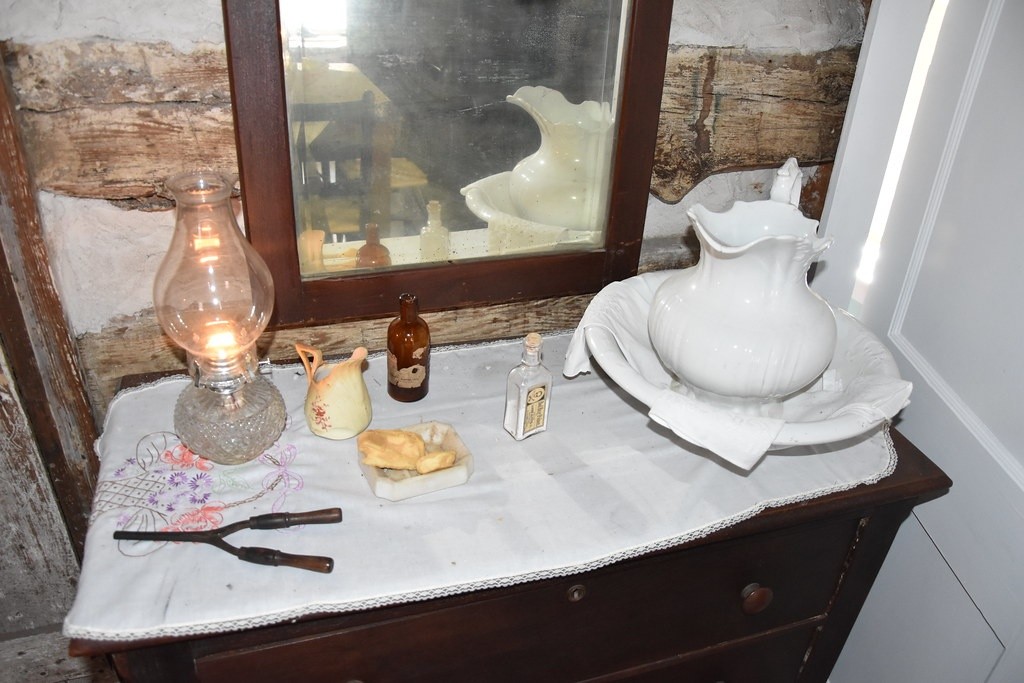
585 270 900 450
465 171 600 241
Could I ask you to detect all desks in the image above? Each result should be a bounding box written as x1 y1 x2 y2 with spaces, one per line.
70 323 953 683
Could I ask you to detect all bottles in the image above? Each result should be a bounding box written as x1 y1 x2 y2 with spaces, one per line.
355 223 391 267
504 332 553 440
419 200 450 262
387 294 431 403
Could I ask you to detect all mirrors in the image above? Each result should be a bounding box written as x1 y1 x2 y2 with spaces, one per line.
222 0 673 332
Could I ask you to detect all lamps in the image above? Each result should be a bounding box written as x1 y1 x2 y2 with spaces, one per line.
164 173 285 464
